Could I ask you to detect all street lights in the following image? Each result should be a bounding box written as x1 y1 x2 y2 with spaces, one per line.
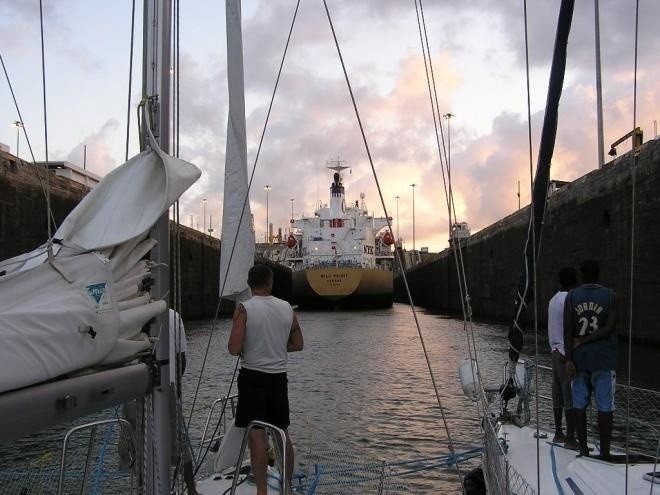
13 121 23 157
396 196 400 241
201 199 208 232
409 184 417 252
263 186 271 243
290 199 294 223
442 114 454 234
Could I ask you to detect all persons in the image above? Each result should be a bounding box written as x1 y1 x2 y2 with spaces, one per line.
228 263 305 495
563 259 621 458
547 265 595 452
117 307 199 495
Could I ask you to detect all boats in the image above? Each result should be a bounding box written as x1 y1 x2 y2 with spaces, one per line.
253 155 395 310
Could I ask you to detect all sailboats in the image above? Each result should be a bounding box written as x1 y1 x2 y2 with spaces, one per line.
457 0 658 495
